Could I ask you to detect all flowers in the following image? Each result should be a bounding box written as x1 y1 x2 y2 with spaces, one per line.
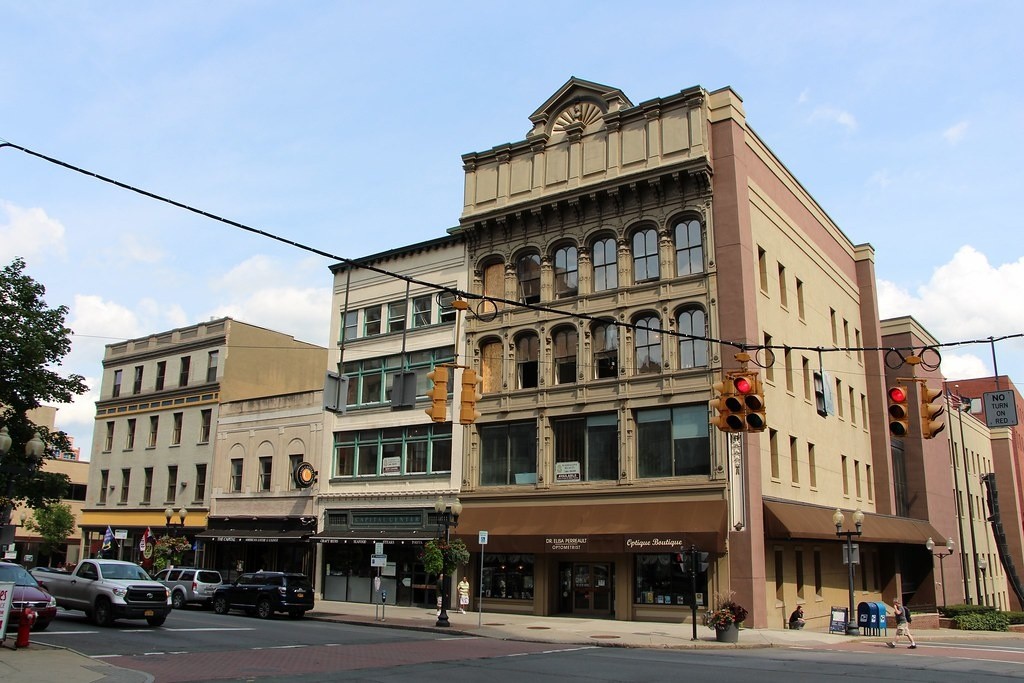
704 590 748 631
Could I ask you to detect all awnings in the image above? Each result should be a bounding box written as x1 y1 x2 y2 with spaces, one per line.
761 500 947 545
307 530 444 546
448 498 728 553
195 529 314 542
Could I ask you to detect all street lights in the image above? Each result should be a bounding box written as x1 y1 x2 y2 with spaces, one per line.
0 425 46 522
927 537 956 609
978 556 989 606
165 505 186 567
8 511 26 543
435 496 463 627
832 507 864 636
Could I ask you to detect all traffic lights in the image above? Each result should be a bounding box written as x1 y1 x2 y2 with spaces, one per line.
709 372 765 433
696 552 709 572
425 366 447 421
461 369 484 424
674 551 690 574
921 380 946 441
888 385 909 437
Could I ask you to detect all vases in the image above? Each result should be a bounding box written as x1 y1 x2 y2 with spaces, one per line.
716 621 738 643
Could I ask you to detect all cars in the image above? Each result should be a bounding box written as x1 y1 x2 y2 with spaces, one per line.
0 561 57 630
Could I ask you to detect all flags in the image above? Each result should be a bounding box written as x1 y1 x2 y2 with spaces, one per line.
102 526 115 551
136 526 156 552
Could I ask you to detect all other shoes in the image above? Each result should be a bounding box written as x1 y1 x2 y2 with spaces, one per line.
886 641 895 648
436 611 440 616
458 607 466 615
907 645 917 649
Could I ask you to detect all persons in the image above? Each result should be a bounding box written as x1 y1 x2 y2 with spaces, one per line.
436 574 448 617
789 606 805 631
885 599 917 649
457 576 470 615
497 578 506 597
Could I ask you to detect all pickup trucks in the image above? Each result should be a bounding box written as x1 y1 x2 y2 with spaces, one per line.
29 558 174 627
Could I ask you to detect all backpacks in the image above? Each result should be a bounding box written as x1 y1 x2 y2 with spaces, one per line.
904 606 912 623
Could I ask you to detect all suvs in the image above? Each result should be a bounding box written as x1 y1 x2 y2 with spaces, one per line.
213 571 316 619
153 568 223 609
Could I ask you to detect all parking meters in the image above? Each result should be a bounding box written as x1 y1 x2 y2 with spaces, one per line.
381 591 386 621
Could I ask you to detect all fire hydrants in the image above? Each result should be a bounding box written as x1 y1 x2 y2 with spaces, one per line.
15 605 39 648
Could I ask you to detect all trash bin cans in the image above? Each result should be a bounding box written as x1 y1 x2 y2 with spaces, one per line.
857 601 887 637
